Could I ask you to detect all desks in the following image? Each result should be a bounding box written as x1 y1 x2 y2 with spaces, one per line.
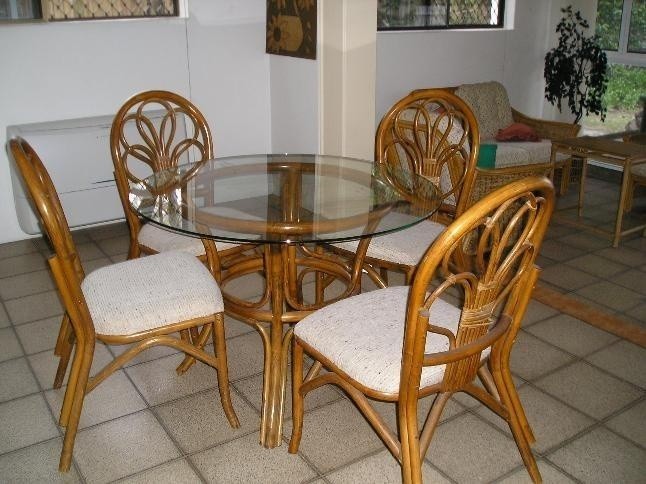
546 129 646 249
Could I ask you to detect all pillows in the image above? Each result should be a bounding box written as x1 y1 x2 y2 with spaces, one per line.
496 120 543 144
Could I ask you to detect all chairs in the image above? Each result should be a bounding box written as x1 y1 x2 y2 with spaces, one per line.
420 79 582 196
